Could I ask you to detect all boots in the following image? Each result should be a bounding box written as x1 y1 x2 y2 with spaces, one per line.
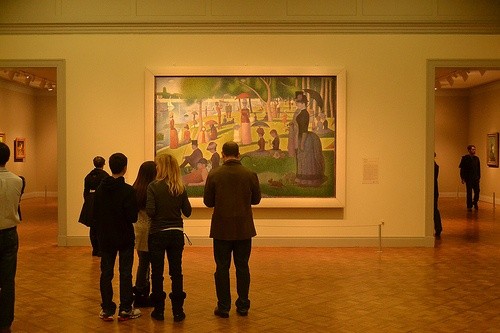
169 292 186 322
150 291 166 320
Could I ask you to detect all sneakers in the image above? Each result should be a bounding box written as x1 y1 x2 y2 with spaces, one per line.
117 309 142 321
99 311 114 321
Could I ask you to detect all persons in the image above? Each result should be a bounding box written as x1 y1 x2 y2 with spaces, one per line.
0 143 22 333
83 153 192 322
203 142 262 317
460 144 481 213
434 152 443 236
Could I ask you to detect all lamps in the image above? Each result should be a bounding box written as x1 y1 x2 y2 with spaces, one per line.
25 75 32 87
478 69 486 77
446 76 454 87
47 83 54 92
458 70 469 81
435 80 442 92
8 70 17 81
39 79 46 89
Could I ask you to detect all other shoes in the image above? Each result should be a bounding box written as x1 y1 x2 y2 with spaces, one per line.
214 308 229 318
236 308 249 316
467 202 478 209
435 231 440 240
133 296 152 308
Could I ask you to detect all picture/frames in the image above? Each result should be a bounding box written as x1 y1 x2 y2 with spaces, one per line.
15 137 26 159
485 131 499 167
143 65 347 210
0 131 6 144
